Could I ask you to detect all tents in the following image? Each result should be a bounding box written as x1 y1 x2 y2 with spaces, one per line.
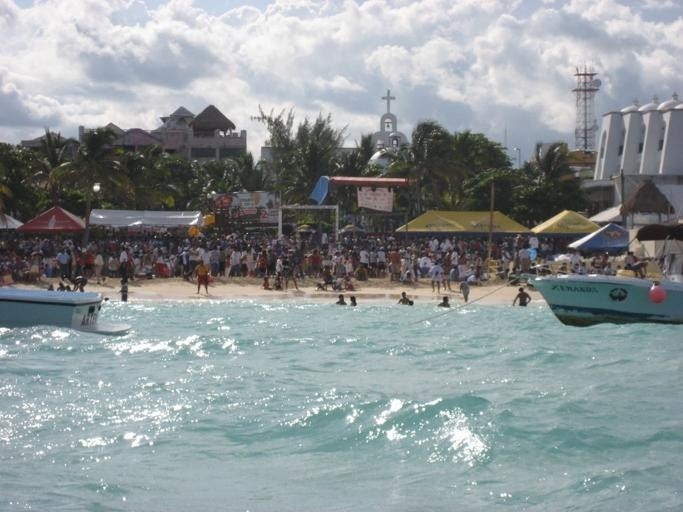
566 222 629 252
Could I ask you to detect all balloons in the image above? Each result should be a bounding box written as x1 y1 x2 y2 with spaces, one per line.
649 287 666 304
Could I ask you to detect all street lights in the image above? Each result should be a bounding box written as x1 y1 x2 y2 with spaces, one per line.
512 146 523 167
93 183 101 209
203 186 216 211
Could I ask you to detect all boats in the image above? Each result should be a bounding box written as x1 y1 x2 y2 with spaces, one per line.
0 286 133 334
525 273 683 327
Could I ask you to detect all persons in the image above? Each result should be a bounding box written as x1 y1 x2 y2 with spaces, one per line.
0 227 651 306
